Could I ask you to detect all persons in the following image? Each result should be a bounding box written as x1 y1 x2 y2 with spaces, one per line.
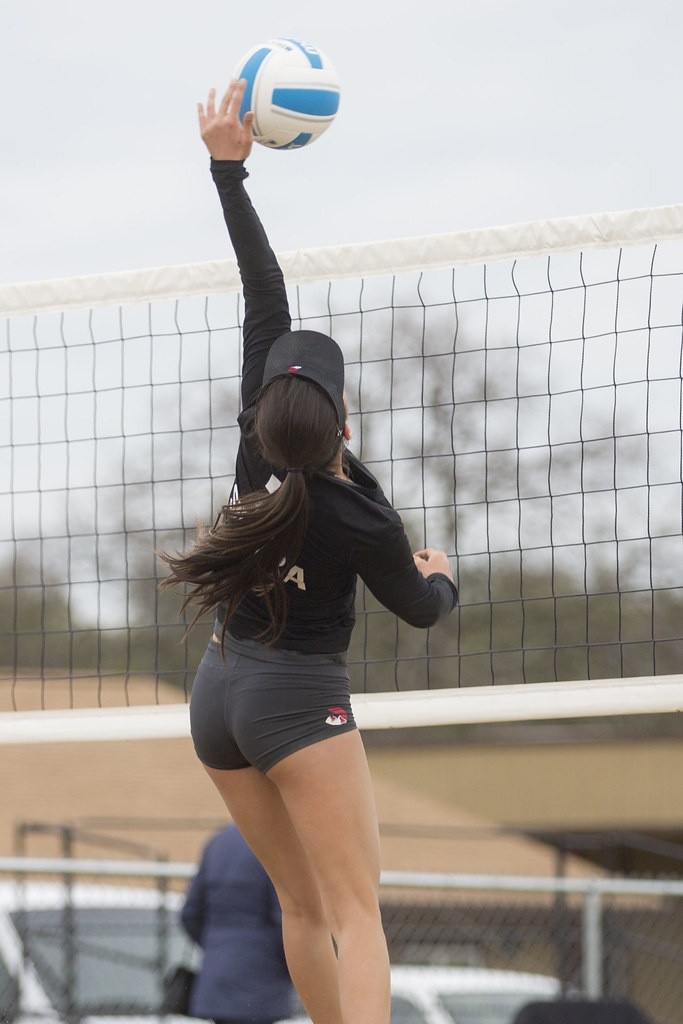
180 828 299 1022
159 76 461 1024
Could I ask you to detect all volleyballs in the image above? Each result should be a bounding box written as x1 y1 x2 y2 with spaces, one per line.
232 37 341 152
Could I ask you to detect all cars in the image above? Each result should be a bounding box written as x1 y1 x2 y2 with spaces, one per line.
1 879 561 1024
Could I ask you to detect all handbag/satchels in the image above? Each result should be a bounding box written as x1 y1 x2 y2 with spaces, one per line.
155 939 201 1018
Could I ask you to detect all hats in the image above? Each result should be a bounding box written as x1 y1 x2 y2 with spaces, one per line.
257 330 345 459
